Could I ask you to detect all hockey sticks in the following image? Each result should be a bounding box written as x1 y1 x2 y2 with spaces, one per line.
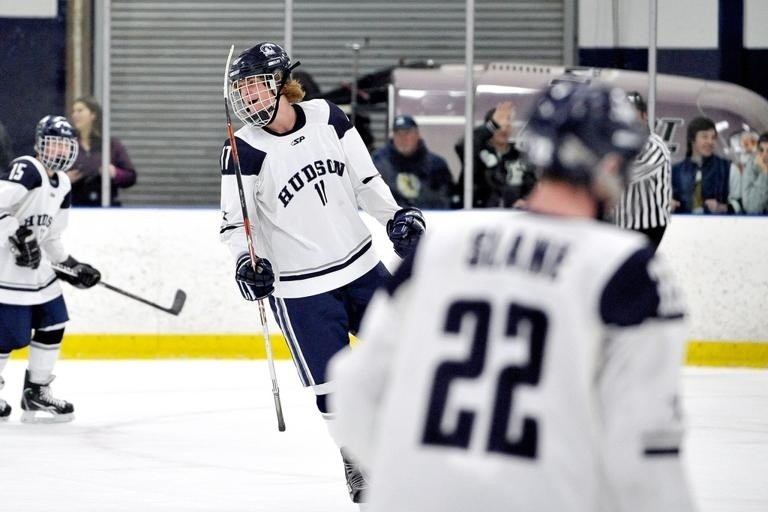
223 44 286 433
9 234 186 315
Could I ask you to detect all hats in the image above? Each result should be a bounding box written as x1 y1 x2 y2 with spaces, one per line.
393 115 416 133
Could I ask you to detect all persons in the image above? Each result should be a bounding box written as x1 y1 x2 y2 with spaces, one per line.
0 115 100 416
67 98 136 205
325 70 694 511
218 41 425 507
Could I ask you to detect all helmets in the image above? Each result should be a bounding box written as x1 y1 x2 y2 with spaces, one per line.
228 40 290 129
527 74 649 184
35 114 80 172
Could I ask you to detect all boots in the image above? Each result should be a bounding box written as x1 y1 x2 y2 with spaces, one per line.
339 448 369 505
20 367 74 415
0 378 12 417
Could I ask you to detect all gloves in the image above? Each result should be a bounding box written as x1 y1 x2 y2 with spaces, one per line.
236 255 275 301
386 207 426 259
7 225 39 269
54 255 101 289
